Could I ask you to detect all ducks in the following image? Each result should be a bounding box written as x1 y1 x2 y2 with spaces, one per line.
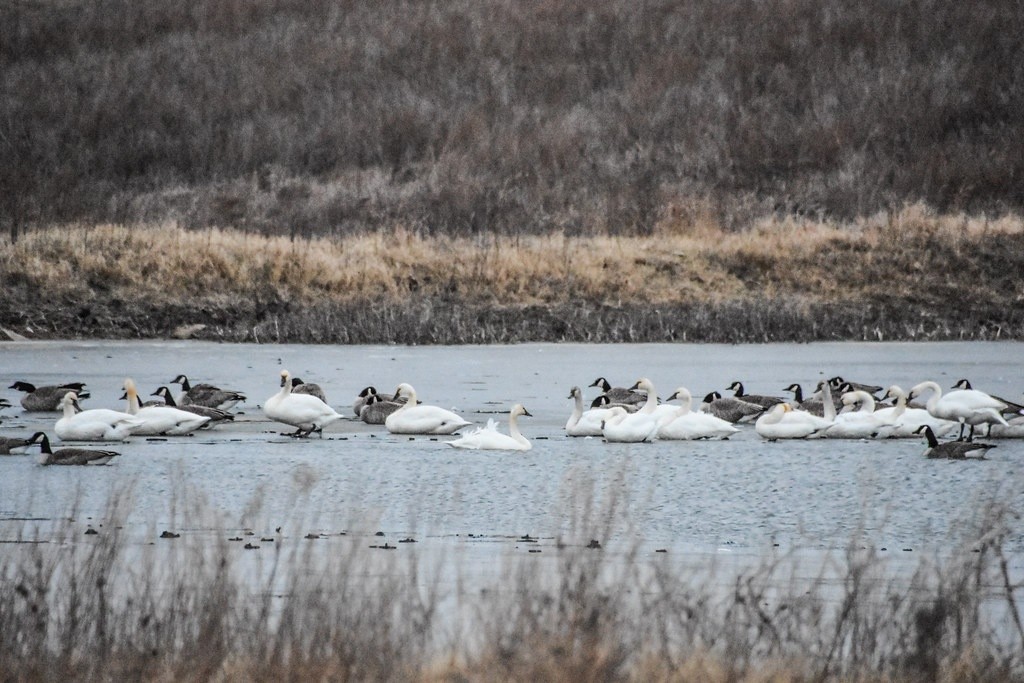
0 369 1023 468
